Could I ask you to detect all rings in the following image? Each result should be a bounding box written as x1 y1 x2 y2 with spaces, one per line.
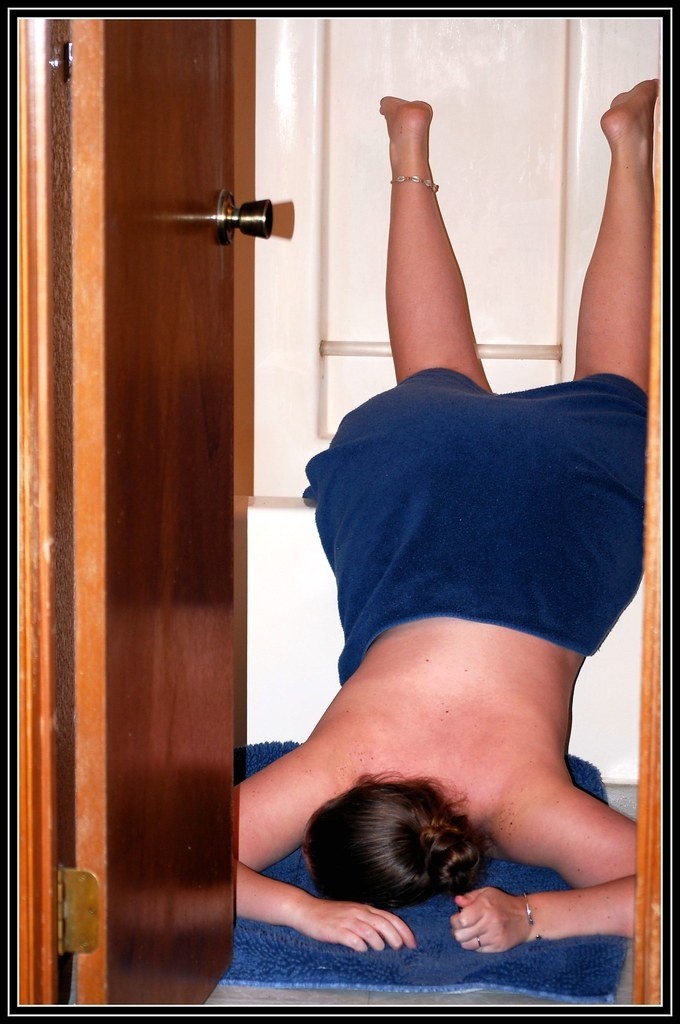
476 936 481 947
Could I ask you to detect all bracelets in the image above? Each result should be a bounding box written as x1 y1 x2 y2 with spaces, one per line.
522 892 541 941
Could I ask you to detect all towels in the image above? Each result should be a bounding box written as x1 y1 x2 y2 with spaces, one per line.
301 366 649 689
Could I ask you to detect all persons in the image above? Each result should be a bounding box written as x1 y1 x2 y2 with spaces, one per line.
233 77 659 953
